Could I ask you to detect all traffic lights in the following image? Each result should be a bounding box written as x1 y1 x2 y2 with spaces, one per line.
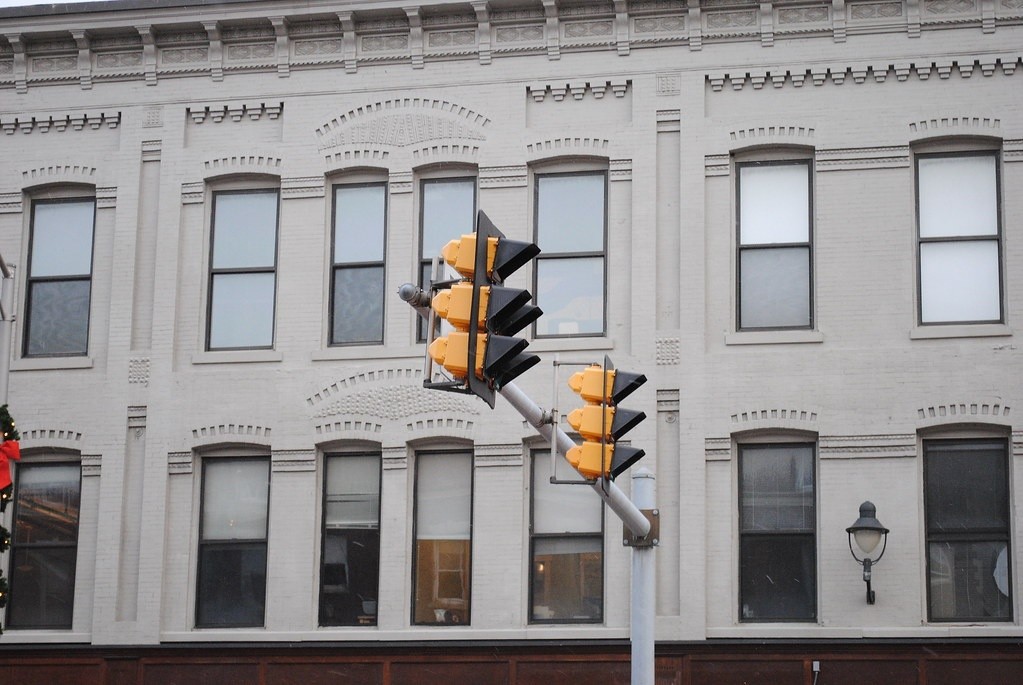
429 211 545 409
567 354 650 487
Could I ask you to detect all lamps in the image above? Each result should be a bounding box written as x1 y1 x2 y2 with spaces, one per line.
845 501 889 605
